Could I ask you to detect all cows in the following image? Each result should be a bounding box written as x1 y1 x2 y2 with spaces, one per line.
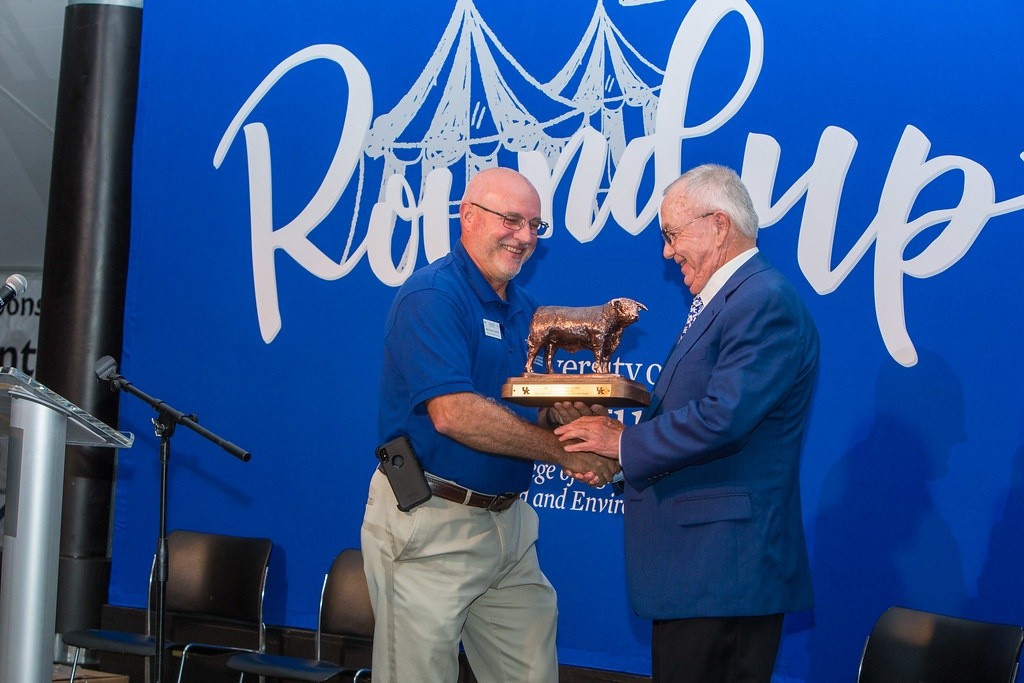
525 298 648 374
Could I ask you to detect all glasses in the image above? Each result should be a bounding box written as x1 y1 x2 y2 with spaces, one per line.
660 212 713 246
471 202 550 237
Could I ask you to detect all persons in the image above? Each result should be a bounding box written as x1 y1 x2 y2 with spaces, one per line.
359 163 622 683
554 163 820 683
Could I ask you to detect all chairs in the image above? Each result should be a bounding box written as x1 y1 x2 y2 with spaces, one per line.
857 606 1024 683
61 528 273 683
226 548 375 683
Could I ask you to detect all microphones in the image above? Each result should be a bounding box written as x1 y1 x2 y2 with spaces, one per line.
0 274 28 309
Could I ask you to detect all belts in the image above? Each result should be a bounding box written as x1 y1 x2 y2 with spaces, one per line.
378 461 520 512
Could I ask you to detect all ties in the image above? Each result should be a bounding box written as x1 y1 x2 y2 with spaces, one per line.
675 296 703 349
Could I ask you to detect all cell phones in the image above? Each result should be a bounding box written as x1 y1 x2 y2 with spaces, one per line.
377 437 432 511
